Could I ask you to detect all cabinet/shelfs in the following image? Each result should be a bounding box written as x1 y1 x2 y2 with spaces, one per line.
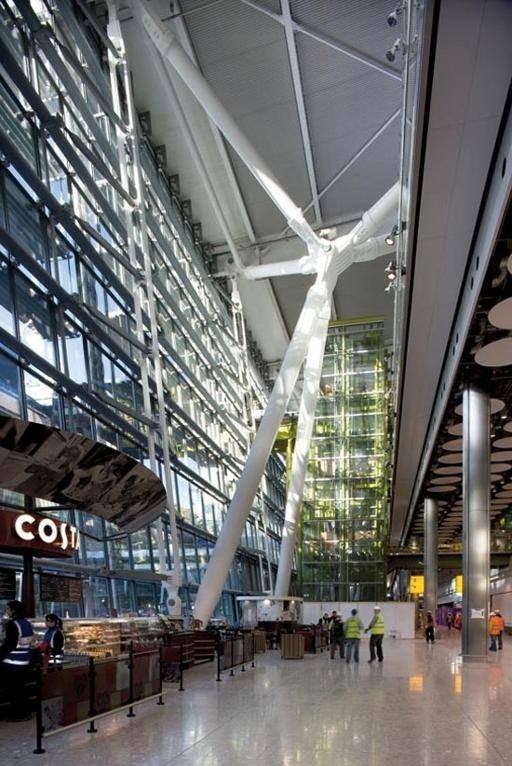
32 613 217 671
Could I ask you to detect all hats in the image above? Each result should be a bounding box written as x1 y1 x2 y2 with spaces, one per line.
375 606 379 609
489 609 500 615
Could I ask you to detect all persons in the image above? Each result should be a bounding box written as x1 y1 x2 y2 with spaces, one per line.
330 618 345 659
322 613 329 630
488 612 500 651
494 609 504 650
15 445 155 523
317 618 330 651
456 615 462 627
328 611 340 623
0 600 34 722
424 610 435 644
343 609 364 664
364 606 384 663
446 612 454 630
43 614 65 672
